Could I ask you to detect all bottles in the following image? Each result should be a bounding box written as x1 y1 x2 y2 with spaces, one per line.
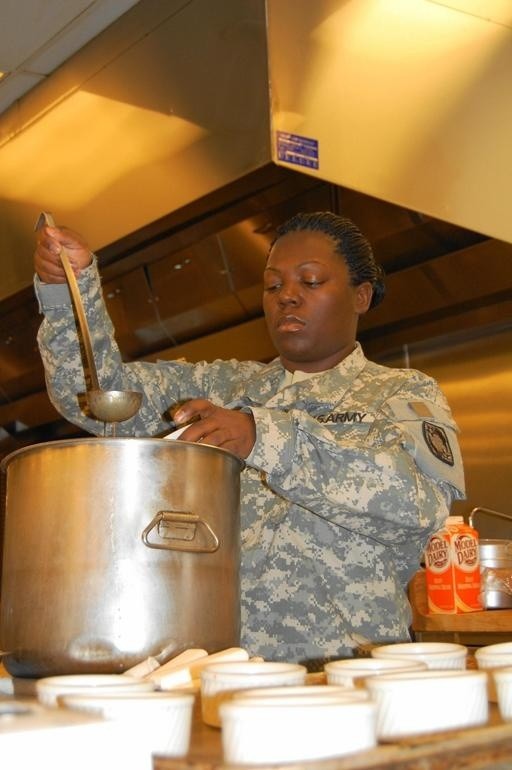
468 507 511 614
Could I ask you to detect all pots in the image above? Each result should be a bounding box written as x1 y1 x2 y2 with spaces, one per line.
3 439 243 676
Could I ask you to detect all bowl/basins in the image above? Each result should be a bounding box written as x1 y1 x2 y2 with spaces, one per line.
370 643 468 671
324 657 430 689
366 670 490 742
34 675 152 712
54 693 195 760
0 698 153 770
494 666 510 727
217 686 377 768
473 643 511 704
204 662 306 729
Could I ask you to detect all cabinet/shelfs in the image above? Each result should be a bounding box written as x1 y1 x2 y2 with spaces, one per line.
1 1 512 458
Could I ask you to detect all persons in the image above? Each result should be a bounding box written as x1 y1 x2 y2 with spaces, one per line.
33 211 466 673
